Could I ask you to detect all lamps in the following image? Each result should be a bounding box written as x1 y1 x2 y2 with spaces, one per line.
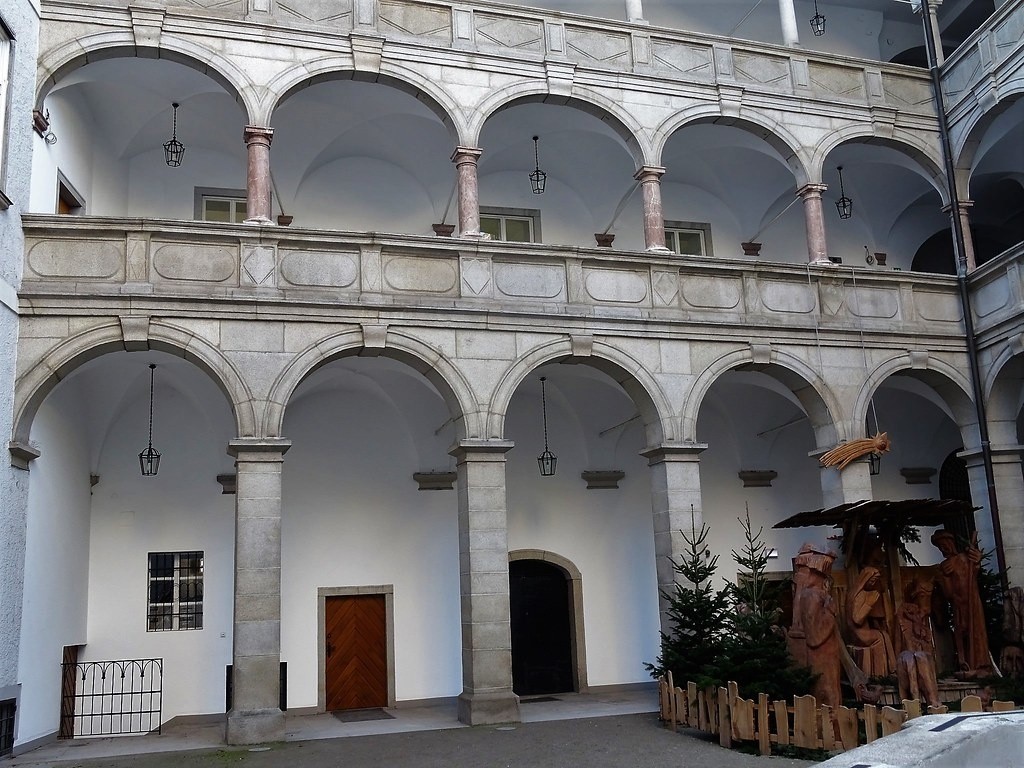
163 103 185 167
835 166 853 219
537 377 558 476
866 415 881 475
138 363 162 476
528 136 547 194
810 0 826 36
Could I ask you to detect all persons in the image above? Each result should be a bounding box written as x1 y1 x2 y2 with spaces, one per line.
790 545 883 740
847 567 898 677
898 584 943 707
931 529 990 670
